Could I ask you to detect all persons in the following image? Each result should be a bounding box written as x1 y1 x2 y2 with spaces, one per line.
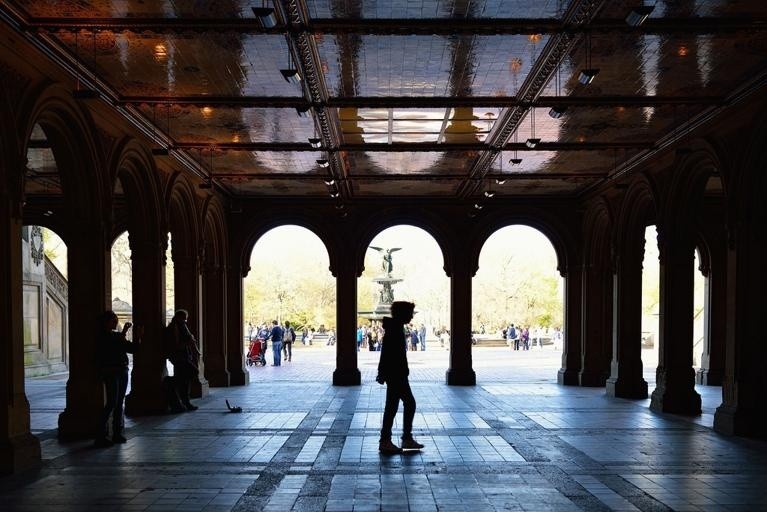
246 318 530 367
376 301 424 452
97 311 145 446
170 310 201 412
382 248 394 277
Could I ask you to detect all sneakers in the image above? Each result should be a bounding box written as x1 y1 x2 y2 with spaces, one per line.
187 403 199 410
401 440 424 449
96 435 127 448
379 442 402 452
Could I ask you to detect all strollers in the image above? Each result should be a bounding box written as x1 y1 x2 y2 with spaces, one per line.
244 328 271 367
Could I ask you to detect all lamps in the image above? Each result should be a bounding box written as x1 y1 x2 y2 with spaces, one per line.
250 3 282 30
576 18 601 86
622 0 657 27
608 142 630 191
546 56 569 120
72 27 99 100
280 35 303 85
198 148 213 189
306 110 353 225
151 103 170 155
464 107 540 219
